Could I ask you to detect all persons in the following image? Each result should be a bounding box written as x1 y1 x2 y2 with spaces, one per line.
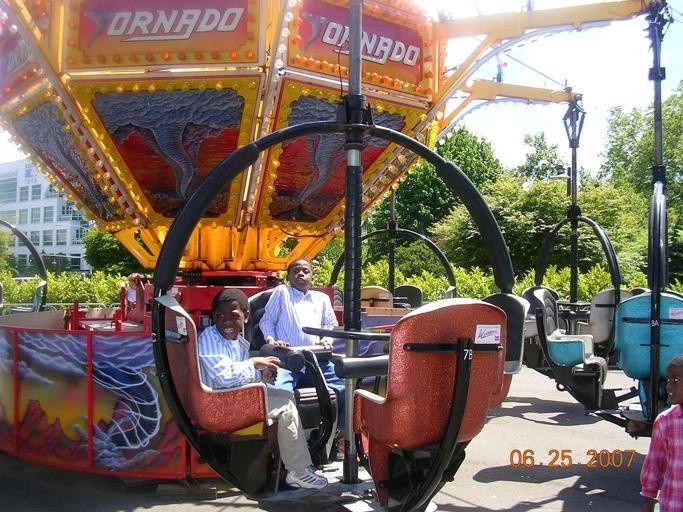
126 274 139 310
257 259 345 463
639 353 683 511
142 273 151 285
196 288 329 491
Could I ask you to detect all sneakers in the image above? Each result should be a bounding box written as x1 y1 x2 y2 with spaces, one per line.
286 471 327 489
